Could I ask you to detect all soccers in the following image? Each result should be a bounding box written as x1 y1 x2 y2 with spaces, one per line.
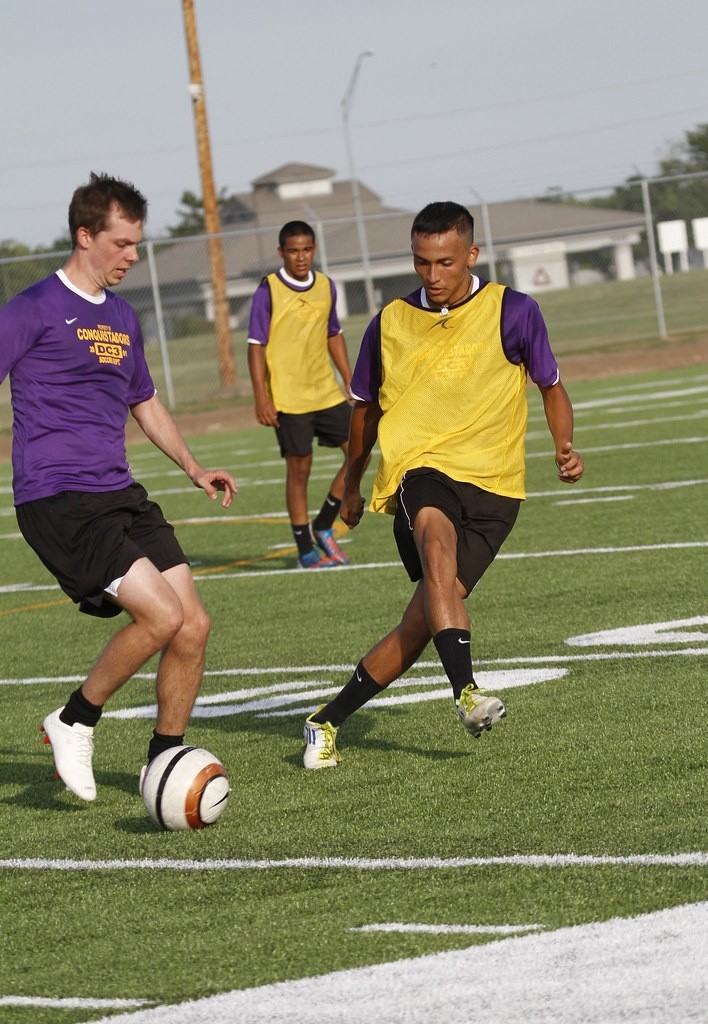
141 744 231 830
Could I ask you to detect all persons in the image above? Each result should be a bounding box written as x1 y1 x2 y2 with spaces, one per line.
302 202 584 768
0 170 239 801
247 220 354 568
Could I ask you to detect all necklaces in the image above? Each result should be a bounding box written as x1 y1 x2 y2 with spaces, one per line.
428 275 472 316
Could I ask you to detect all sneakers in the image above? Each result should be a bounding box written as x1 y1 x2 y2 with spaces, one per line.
138 766 148 794
304 706 344 769
42 706 97 801
457 683 506 738
309 522 349 564
297 547 337 567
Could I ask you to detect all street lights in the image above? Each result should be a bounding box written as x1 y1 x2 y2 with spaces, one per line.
339 48 380 318
